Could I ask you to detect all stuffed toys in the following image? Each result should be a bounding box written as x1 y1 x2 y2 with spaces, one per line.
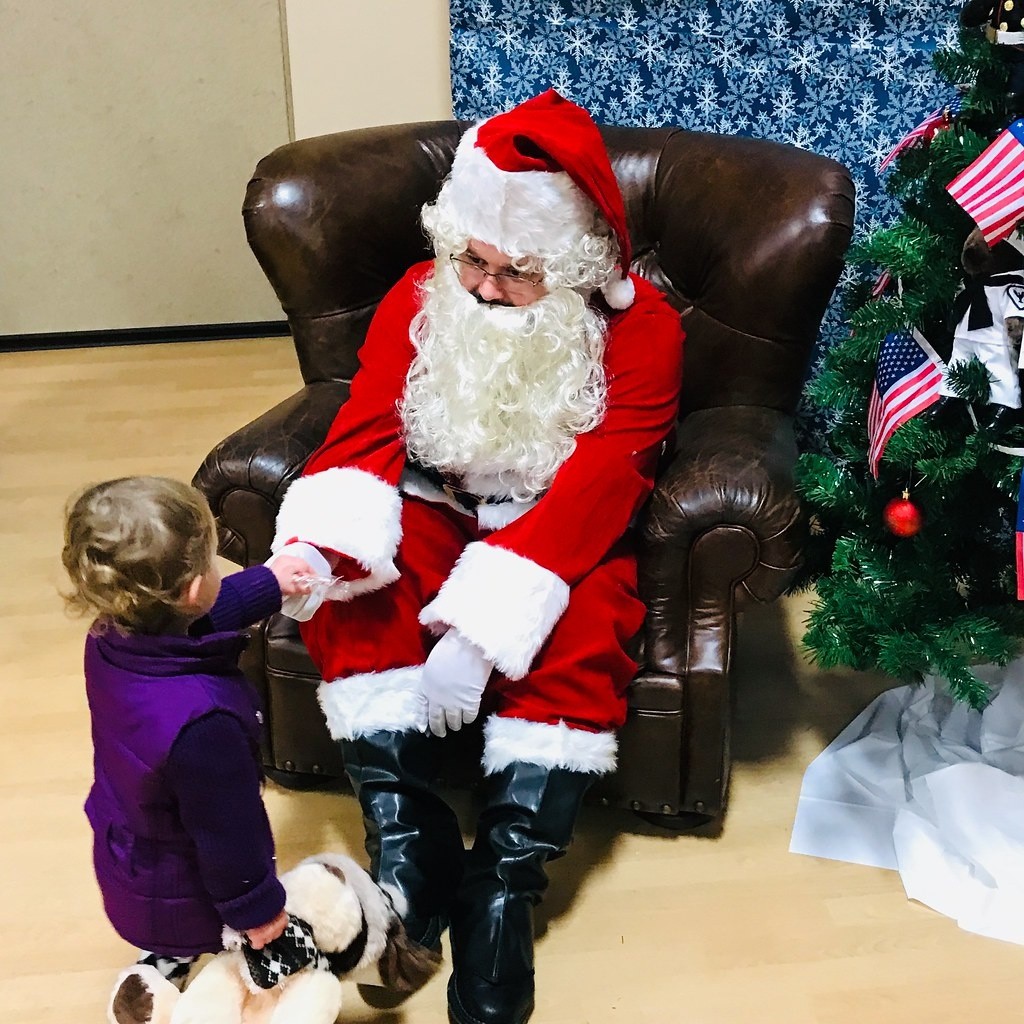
937 221 1024 410
105 849 442 1024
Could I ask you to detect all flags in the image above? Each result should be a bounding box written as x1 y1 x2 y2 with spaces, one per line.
879 91 968 173
865 321 944 478
946 118 1024 248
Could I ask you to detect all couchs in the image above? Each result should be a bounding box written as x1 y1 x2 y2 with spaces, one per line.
188 117 856 827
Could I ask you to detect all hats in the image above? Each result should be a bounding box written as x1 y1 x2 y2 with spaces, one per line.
439 87 635 310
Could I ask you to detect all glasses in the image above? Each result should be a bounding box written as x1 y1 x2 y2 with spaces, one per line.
449 252 545 294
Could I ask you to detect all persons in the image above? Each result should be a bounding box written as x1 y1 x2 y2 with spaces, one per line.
265 90 683 1024
56 473 315 990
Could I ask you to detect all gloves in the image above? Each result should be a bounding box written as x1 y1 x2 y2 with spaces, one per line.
413 626 495 738
263 541 331 622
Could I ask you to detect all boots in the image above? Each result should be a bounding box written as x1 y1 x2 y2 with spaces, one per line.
339 728 465 966
448 760 600 1024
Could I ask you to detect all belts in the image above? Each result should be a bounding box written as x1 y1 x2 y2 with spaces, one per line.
404 450 548 520
